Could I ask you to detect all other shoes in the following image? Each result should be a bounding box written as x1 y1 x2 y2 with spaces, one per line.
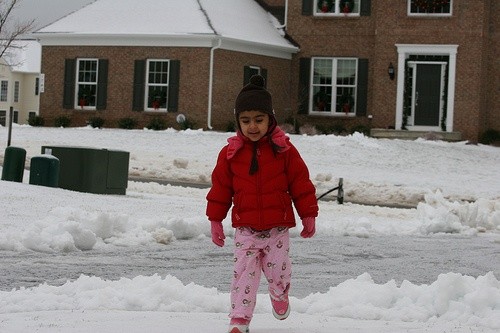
227 318 251 333
271 292 291 320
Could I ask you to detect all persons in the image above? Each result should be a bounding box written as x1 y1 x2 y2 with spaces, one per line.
206 74 319 333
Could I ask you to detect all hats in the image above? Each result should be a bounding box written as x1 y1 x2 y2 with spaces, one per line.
234 74 280 176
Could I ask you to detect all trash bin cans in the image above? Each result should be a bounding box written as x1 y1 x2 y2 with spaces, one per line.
29 156 60 187
1 146 26 182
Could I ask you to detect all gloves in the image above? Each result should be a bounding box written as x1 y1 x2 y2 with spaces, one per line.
300 218 316 238
210 221 226 246
